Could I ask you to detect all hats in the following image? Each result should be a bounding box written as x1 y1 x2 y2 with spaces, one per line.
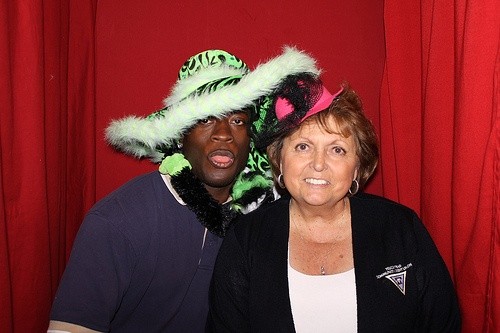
103 45 322 162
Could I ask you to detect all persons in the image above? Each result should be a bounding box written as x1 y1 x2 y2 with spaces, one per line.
47 49 255 333
205 76 461 333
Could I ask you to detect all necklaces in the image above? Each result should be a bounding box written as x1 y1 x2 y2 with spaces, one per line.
288 201 345 275
